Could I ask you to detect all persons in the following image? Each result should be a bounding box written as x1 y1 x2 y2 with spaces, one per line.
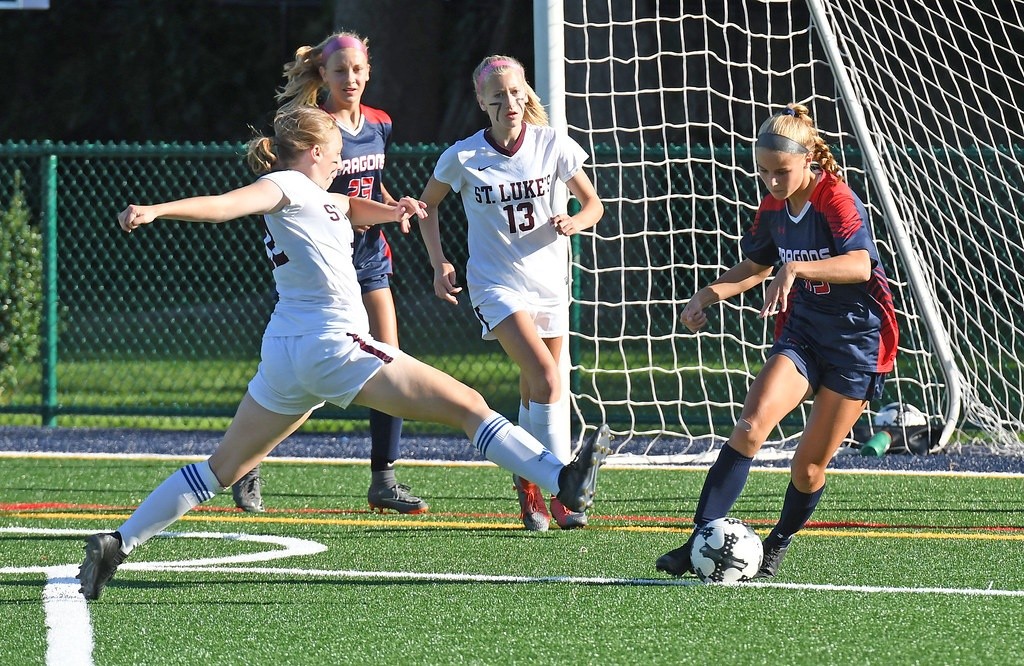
75 107 614 602
231 29 430 514
417 55 606 532
655 103 899 579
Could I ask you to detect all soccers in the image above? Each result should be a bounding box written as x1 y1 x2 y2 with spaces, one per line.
689 516 764 584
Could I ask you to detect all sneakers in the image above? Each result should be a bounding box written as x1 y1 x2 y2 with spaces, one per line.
75 531 128 602
232 469 266 514
368 481 428 514
751 529 792 578
512 476 549 531
550 496 588 530
655 543 697 578
556 423 615 513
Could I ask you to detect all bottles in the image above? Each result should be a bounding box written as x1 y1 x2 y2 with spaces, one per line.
861 431 892 458
